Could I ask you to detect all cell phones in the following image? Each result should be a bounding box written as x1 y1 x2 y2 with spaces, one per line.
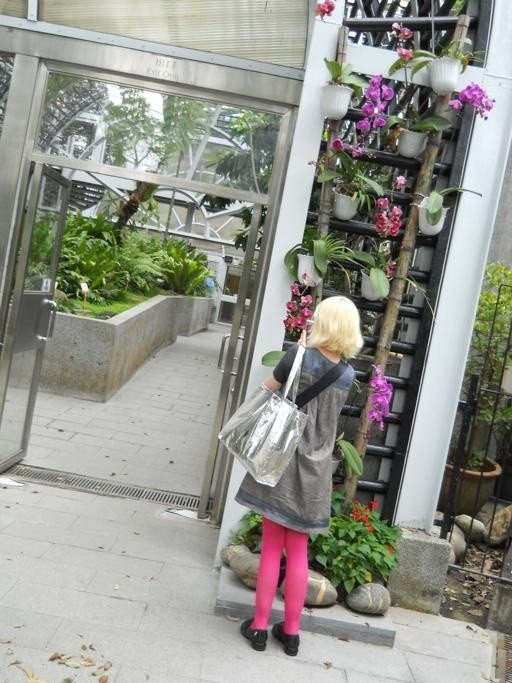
305 320 314 339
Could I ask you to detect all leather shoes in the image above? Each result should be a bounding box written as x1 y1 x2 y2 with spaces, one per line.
240 618 268 650
273 622 300 656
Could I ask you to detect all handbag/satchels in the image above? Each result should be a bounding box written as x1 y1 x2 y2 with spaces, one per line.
218 383 309 488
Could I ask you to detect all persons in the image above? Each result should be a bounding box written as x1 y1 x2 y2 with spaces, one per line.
229 294 367 653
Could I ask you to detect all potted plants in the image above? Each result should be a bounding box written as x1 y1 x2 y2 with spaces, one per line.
441 258 512 522
